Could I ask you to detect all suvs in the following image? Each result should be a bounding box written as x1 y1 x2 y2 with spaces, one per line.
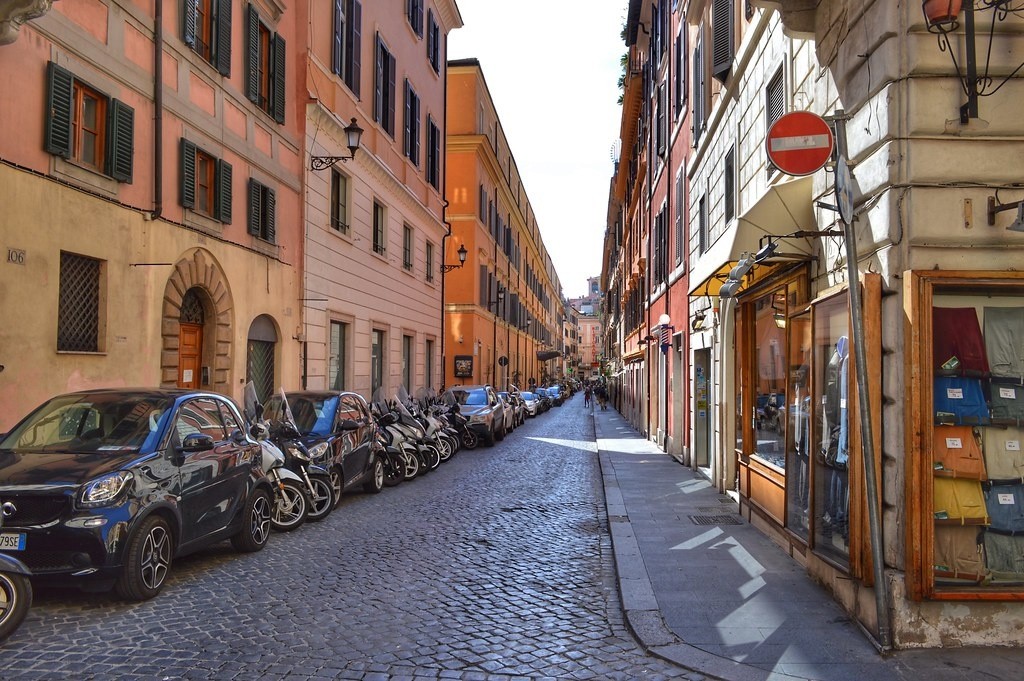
438 384 504 446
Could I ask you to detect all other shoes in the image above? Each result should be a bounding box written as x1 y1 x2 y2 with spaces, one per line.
588 405 589 407
584 405 586 407
604 406 607 409
601 406 603 410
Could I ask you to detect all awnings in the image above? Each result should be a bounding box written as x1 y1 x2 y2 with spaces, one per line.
688 176 814 296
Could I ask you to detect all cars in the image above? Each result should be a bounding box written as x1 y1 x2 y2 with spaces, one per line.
0 387 280 601
248 390 387 509
495 387 564 436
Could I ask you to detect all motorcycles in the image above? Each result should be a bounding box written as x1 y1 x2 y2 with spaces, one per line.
240 380 335 533
366 383 479 487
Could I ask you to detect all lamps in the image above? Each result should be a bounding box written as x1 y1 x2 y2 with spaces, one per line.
692 294 712 331
440 244 468 272
488 286 504 304
659 313 675 354
988 194 1024 232
729 251 821 282
753 229 845 263
310 116 364 172
644 336 655 340
637 340 648 344
719 280 744 297
771 292 786 329
520 315 532 329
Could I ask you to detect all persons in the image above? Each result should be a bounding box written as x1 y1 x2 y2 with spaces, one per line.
570 386 574 398
584 381 608 410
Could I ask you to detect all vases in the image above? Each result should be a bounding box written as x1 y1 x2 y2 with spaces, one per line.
922 1 962 33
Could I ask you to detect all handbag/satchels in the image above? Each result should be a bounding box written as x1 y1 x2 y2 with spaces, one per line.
586 395 590 399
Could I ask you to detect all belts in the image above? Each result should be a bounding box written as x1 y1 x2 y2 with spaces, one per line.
984 478 1023 486
935 567 986 580
983 525 1024 538
991 376 1024 383
992 418 1022 429
935 469 987 482
935 414 990 424
933 367 985 379
991 568 1023 580
935 517 994 526
